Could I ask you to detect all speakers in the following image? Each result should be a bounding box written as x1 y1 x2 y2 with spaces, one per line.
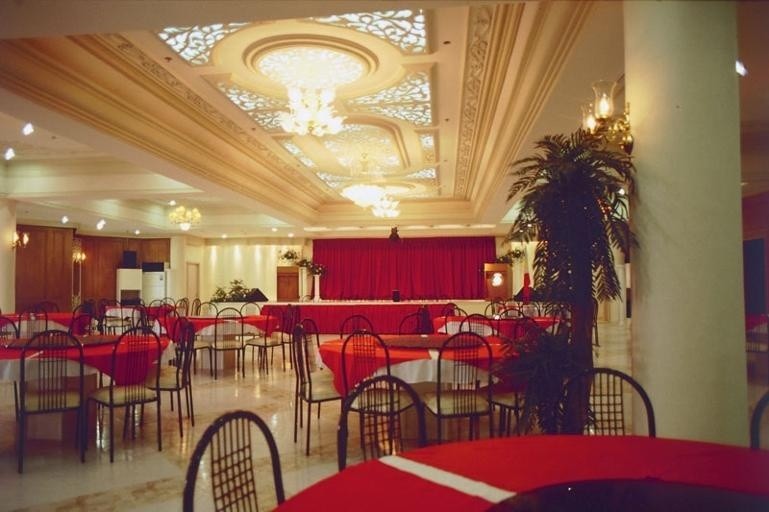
123 250 137 267
392 288 400 302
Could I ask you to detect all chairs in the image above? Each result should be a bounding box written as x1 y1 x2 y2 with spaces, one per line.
751 390 769 449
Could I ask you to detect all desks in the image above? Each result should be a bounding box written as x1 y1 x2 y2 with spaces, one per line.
268 435 769 511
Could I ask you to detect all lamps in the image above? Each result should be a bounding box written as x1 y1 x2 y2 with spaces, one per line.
11 232 28 249
581 79 634 155
168 203 200 231
239 32 401 220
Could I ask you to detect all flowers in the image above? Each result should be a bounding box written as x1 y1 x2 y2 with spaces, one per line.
284 250 326 276
